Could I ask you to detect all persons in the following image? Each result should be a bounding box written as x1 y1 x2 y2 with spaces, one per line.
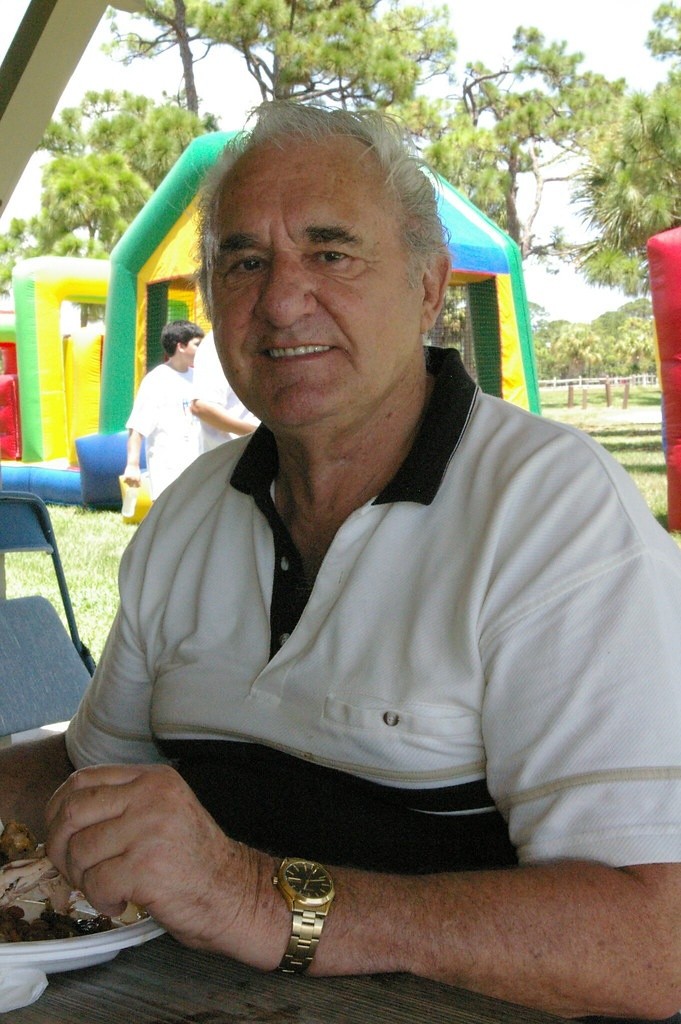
120 318 203 500
37 101 681 1021
189 332 262 457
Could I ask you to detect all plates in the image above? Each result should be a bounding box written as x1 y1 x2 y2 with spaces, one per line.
0 892 169 975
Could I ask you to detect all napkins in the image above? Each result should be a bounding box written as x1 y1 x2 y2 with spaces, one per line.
0 967 50 1015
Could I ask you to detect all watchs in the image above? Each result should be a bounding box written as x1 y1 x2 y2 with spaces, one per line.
270 857 335 976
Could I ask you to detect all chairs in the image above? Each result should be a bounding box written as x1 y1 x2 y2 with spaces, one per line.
0 490 99 736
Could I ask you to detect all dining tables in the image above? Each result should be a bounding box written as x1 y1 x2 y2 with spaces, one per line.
0 932 586 1024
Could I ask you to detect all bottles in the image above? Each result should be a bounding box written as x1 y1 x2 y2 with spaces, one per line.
122 487 137 517
